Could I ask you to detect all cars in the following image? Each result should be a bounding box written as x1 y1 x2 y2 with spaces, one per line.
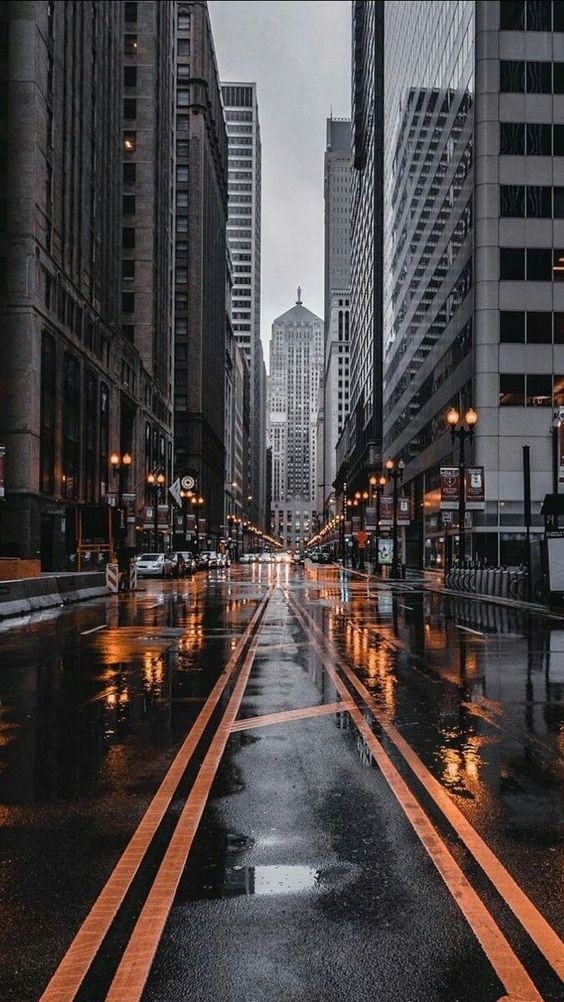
135 549 333 580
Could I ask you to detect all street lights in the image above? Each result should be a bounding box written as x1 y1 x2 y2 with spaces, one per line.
147 472 165 553
369 476 387 575
109 451 133 591
354 490 369 570
226 514 282 564
385 456 406 579
190 496 204 556
179 489 193 551
447 407 478 569
